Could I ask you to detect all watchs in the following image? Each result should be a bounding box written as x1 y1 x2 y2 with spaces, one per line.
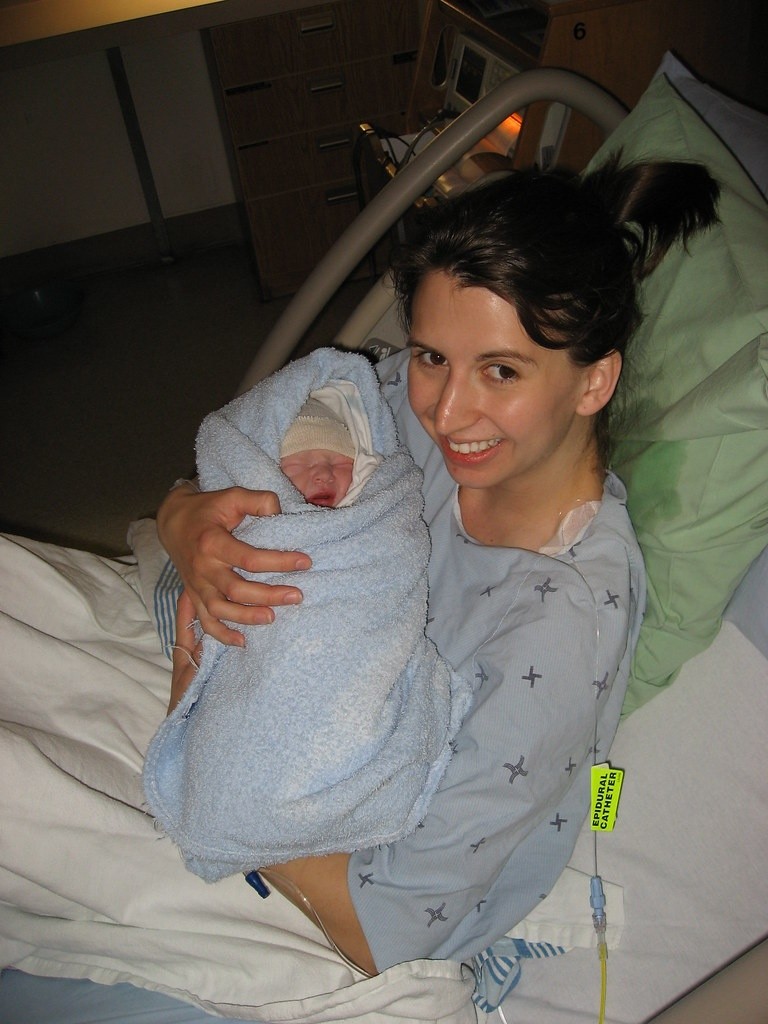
164 478 200 497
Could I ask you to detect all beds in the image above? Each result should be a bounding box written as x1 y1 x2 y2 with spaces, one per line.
236 53 768 1024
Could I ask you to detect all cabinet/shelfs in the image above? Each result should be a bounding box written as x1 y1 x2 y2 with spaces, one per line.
200 0 418 301
355 1 716 279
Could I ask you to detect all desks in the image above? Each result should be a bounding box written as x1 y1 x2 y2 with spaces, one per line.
0 0 323 264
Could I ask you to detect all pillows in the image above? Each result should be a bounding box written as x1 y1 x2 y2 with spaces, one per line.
575 72 768 722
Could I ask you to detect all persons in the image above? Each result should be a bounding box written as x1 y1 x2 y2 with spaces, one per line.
0 160 723 982
141 343 472 884
